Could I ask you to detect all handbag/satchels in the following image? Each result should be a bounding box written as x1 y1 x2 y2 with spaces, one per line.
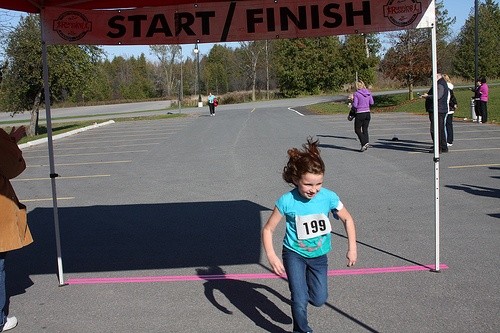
347 107 357 121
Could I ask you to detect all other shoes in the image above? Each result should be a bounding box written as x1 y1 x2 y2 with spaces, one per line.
447 142 453 147
431 149 441 154
441 148 448 152
361 143 369 151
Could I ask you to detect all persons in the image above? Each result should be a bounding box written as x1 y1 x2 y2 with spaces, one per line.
477 75 488 124
352 79 374 152
469 79 482 123
419 70 448 153
262 134 357 333
0 125 35 333
441 73 454 143
207 92 216 116
446 88 458 146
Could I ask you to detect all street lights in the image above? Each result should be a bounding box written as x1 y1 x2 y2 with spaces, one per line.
194 44 203 108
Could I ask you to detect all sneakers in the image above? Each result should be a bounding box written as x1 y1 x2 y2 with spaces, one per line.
3 316 17 331
